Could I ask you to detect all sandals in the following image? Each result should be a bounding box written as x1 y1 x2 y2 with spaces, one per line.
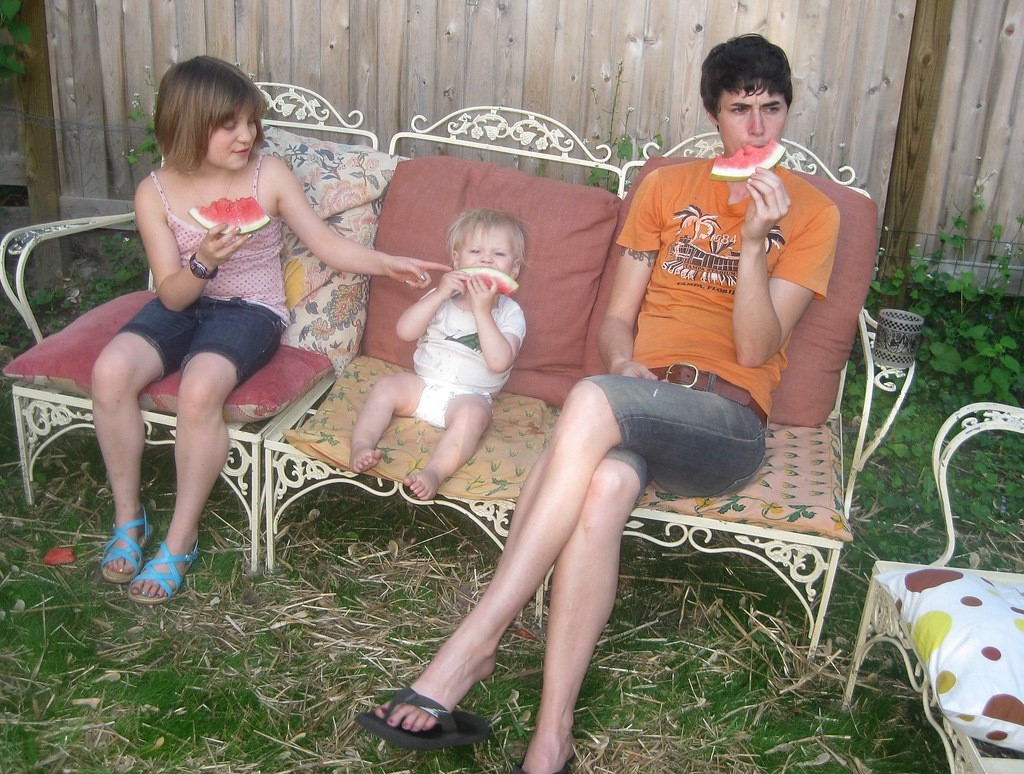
100 503 153 583
127 540 199 605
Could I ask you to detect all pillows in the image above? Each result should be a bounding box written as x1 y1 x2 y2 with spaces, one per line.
360 155 624 408
256 125 399 374
582 155 880 430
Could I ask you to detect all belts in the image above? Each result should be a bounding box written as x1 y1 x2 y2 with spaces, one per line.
648 362 766 429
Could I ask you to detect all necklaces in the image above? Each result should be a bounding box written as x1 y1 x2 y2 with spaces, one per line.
187 173 235 206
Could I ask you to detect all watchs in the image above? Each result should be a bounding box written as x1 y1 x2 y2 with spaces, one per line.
190 252 218 279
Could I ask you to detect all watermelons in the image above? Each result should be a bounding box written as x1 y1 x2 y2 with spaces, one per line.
189 198 270 235
709 140 786 181
455 266 520 298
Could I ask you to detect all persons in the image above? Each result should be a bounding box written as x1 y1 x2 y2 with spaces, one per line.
350 209 528 501
90 55 453 604
357 33 841 773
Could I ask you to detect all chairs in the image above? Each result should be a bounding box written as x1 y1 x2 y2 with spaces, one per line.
0 82 917 661
840 401 1024 774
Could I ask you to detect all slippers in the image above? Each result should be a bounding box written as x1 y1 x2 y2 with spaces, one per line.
510 753 576 774
354 687 489 749
